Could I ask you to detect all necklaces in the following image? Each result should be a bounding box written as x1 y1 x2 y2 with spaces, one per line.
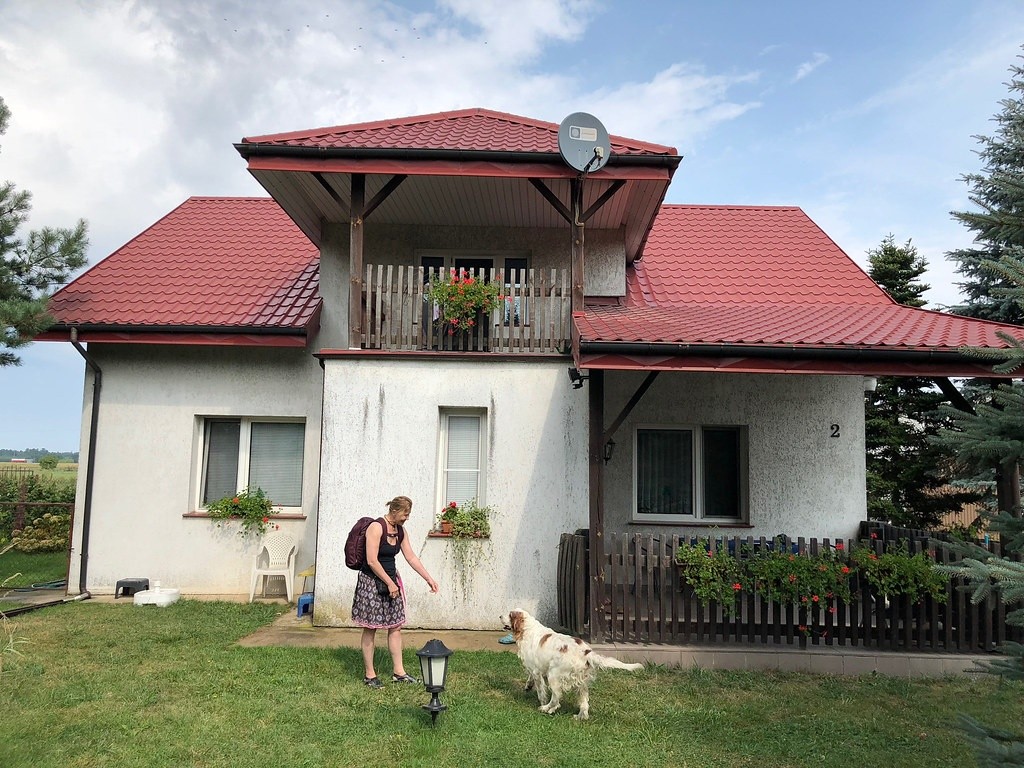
385 516 397 530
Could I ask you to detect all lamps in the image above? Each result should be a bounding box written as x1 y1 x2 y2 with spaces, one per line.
603 438 617 467
566 367 589 390
414 639 454 696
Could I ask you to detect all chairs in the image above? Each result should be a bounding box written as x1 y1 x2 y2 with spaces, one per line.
631 536 673 595
249 530 299 603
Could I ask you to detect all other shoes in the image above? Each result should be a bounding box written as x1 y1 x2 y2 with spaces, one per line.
499 632 518 644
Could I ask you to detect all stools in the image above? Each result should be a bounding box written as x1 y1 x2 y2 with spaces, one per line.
297 593 313 618
114 577 150 599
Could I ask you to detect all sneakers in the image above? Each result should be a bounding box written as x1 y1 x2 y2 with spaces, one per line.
392 674 422 684
364 677 386 690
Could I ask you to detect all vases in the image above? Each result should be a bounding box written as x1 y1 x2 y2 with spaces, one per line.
426 311 488 352
441 520 454 533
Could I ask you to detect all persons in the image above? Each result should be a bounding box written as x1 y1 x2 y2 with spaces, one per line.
343 495 440 687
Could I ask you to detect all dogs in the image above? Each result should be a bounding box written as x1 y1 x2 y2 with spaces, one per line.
499 608 644 718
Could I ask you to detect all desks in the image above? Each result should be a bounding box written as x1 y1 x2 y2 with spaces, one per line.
678 537 800 561
297 564 315 595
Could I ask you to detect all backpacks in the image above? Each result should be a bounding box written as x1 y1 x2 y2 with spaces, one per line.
344 517 404 570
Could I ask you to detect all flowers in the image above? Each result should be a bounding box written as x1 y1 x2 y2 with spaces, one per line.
674 528 950 640
418 496 505 609
423 265 515 338
201 481 284 538
